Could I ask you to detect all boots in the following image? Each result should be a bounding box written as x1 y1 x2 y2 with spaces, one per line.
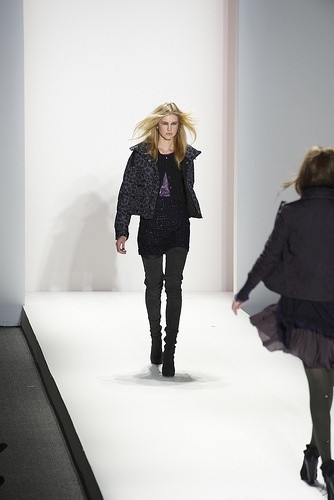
144 273 166 364
163 273 183 377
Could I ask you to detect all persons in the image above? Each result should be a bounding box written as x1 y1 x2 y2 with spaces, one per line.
231 146 334 500
113 102 202 378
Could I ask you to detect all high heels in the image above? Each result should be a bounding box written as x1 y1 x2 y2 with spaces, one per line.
300 444 320 484
320 459 334 500
